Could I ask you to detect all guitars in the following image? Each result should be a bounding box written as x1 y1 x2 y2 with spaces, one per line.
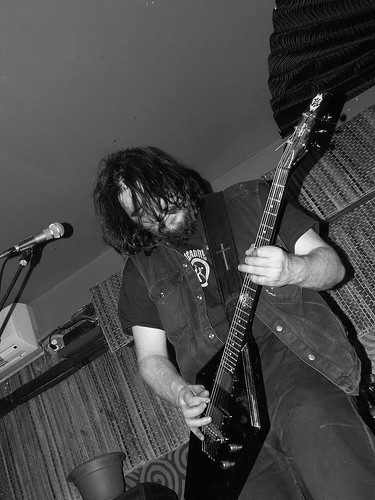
202 94 333 470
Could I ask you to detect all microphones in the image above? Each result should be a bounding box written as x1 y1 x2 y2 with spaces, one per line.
0 222 65 260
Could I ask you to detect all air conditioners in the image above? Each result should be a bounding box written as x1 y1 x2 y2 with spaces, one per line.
0 303 48 383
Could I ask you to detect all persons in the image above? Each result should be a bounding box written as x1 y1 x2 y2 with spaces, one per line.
93 147 375 500
